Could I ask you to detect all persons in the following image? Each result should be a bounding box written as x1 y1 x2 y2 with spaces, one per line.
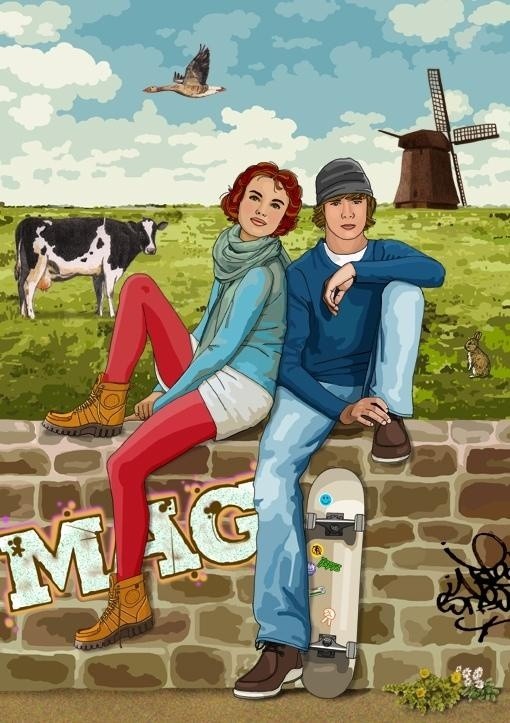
232 159 446 700
43 161 303 652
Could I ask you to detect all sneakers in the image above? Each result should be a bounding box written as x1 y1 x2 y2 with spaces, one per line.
233 640 304 700
372 410 411 463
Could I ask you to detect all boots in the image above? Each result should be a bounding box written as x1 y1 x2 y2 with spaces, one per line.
42 372 130 437
76 572 152 649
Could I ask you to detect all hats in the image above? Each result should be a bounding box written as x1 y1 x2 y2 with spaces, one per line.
317 157 373 203
